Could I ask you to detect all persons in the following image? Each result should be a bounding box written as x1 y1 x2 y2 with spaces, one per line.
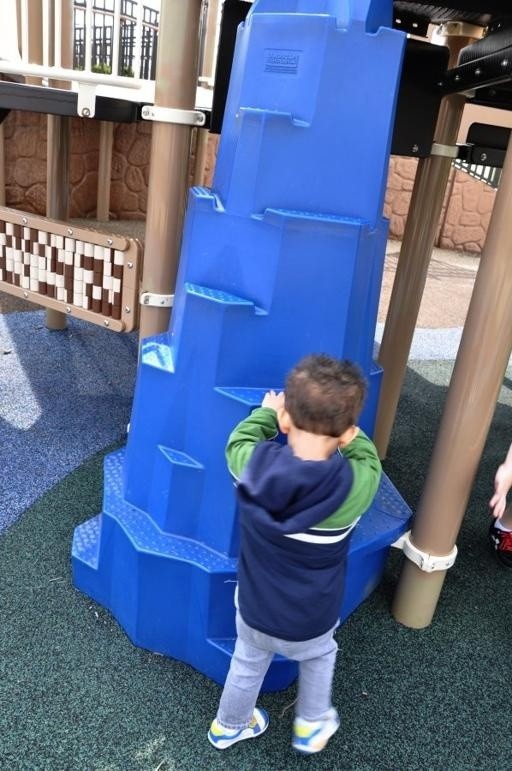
488 442 512 570
207 354 383 754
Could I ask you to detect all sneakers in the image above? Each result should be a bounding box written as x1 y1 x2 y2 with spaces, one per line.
207 708 270 751
289 707 340 756
